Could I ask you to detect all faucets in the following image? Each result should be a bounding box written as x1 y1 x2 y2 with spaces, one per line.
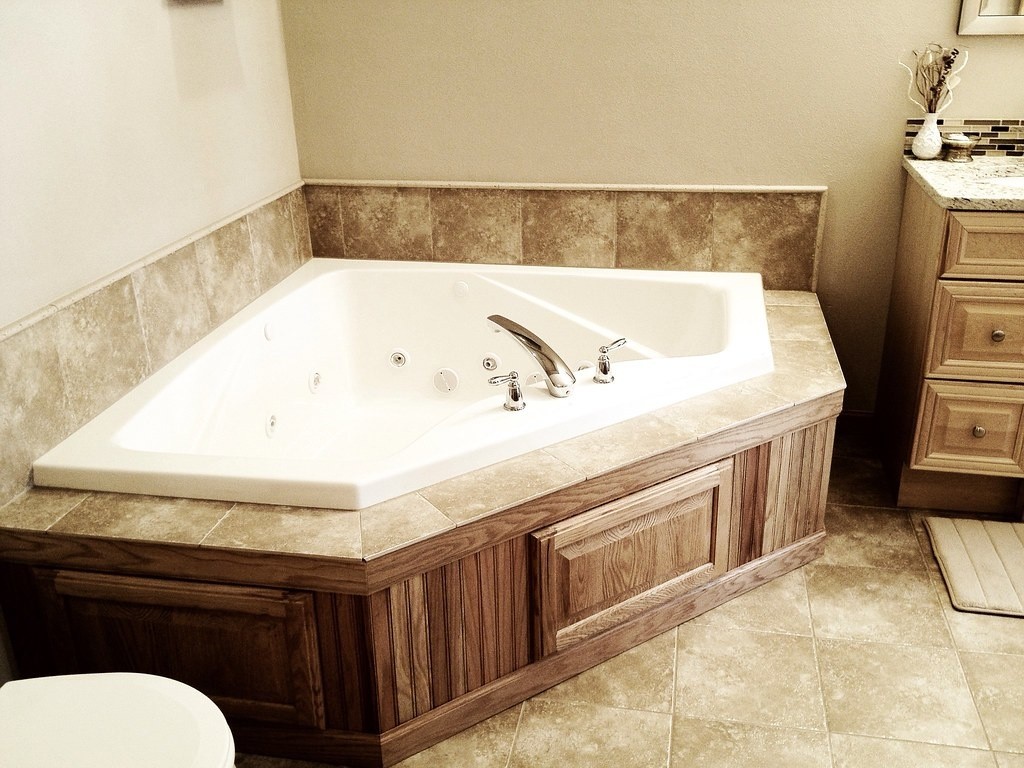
486 314 577 399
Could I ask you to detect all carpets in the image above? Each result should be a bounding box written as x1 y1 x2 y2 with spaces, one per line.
920 517 1023 618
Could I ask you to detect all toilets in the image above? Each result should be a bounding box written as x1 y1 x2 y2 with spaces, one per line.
1 672 235 765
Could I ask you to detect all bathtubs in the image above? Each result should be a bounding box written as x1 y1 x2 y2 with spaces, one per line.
31 254 847 766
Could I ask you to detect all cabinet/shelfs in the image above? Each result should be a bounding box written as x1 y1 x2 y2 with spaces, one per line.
873 174 1024 515
